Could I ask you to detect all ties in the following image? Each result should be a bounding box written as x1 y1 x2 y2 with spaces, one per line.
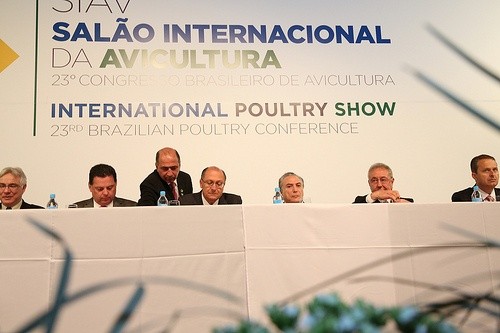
486 195 494 202
169 182 177 200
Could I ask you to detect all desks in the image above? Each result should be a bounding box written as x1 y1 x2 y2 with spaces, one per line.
1 201 500 333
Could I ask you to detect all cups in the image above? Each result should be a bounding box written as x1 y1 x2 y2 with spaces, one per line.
168 200 180 205
65 204 78 208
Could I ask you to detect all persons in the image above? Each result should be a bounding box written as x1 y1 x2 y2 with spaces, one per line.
73 163 137 207
0 167 45 210
137 147 192 206
451 154 500 202
351 163 413 203
279 172 305 203
178 166 242 205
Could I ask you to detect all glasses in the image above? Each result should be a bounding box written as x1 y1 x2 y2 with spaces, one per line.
201 178 224 188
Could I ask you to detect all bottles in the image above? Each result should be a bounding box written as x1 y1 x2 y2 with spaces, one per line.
471 186 482 202
273 187 285 203
157 191 168 205
47 194 58 209
